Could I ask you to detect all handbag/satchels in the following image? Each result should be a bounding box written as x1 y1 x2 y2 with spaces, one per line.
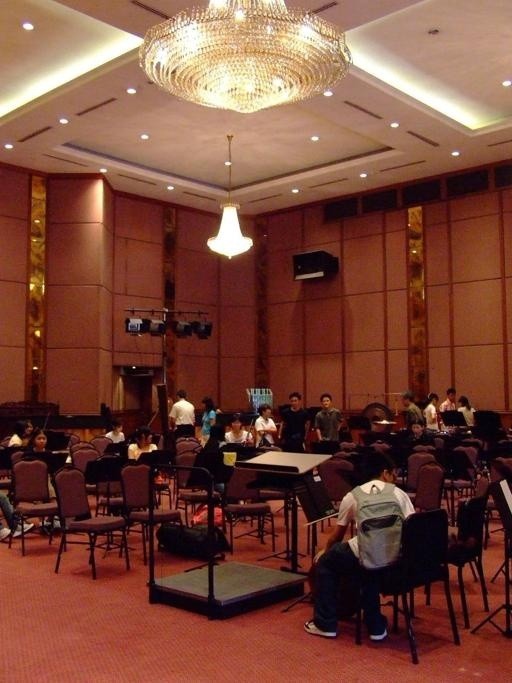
154 520 233 562
190 502 228 528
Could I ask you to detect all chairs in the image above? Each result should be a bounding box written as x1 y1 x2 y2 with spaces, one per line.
1 402 512 665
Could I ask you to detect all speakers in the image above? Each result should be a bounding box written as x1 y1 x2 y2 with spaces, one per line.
293 250 339 280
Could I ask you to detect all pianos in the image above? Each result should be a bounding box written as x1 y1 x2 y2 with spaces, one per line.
191 409 260 427
45 414 110 437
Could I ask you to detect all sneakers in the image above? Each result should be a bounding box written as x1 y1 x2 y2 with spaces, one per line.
0 527 12 541
303 618 338 638
12 521 35 537
367 627 389 641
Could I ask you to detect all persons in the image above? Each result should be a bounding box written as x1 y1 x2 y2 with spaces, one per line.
315 393 343 441
167 390 195 445
201 396 216 439
279 392 311 453
401 394 423 422
224 415 254 447
106 418 125 443
408 419 436 452
255 404 277 448
22 429 53 454
204 423 226 449
438 388 457 432
8 421 33 447
423 393 439 431
304 451 416 641
128 427 158 460
417 391 430 409
457 395 476 433
0 492 34 538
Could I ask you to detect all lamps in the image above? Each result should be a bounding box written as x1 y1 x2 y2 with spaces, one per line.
137 0 354 114
206 133 254 261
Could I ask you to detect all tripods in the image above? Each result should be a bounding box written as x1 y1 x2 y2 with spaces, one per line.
471 528 512 636
85 485 315 613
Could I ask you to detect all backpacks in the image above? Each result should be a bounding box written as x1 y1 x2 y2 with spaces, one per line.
351 482 406 571
